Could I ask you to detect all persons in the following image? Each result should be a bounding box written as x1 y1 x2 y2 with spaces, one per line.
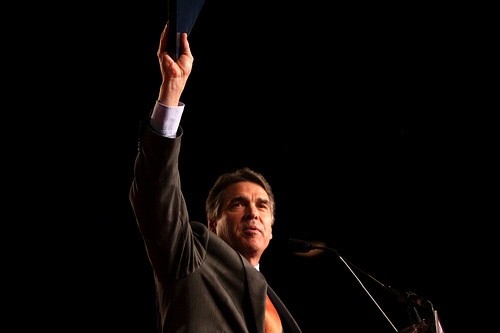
127 18 288 333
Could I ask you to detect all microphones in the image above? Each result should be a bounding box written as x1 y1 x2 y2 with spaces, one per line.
286 238 338 256
